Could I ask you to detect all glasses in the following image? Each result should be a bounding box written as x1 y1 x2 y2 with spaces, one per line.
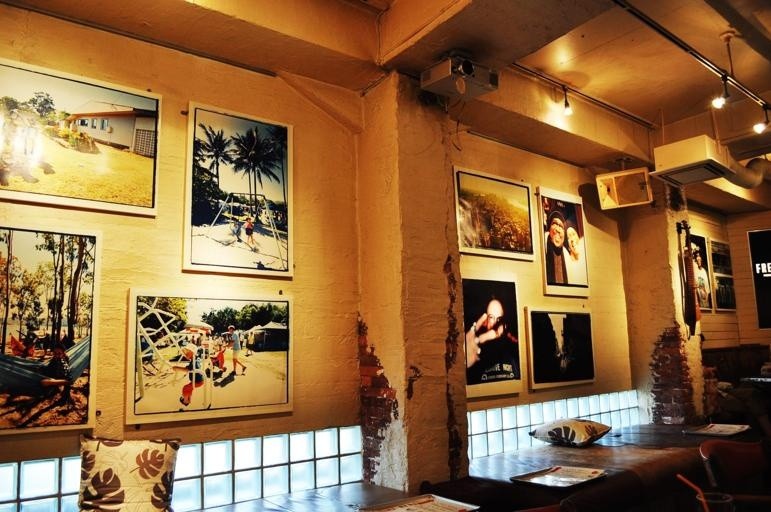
545 222 567 231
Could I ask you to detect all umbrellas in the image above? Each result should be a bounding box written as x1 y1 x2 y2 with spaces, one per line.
177 329 207 336
184 321 214 331
185 329 212 334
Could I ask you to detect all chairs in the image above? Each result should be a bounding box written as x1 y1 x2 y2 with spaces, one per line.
699 438 771 512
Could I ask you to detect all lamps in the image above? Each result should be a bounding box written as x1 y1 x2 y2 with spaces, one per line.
753 103 771 134
711 72 731 110
562 83 574 118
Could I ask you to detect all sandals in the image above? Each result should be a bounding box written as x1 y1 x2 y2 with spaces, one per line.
242 366 251 373
230 370 239 375
179 397 194 406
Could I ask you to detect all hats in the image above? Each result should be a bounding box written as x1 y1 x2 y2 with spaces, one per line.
549 211 568 225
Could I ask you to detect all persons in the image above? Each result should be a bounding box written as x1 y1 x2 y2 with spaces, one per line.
247 332 255 356
39 343 74 385
228 325 247 374
567 226 589 287
465 294 521 387
694 250 711 308
240 333 245 349
172 343 206 407
545 211 580 286
178 333 205 349
242 217 255 245
229 223 241 236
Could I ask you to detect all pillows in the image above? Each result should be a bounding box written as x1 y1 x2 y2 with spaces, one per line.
528 418 611 447
76 433 180 512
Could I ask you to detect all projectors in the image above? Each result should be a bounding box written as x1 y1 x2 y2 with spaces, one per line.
420 56 499 102
